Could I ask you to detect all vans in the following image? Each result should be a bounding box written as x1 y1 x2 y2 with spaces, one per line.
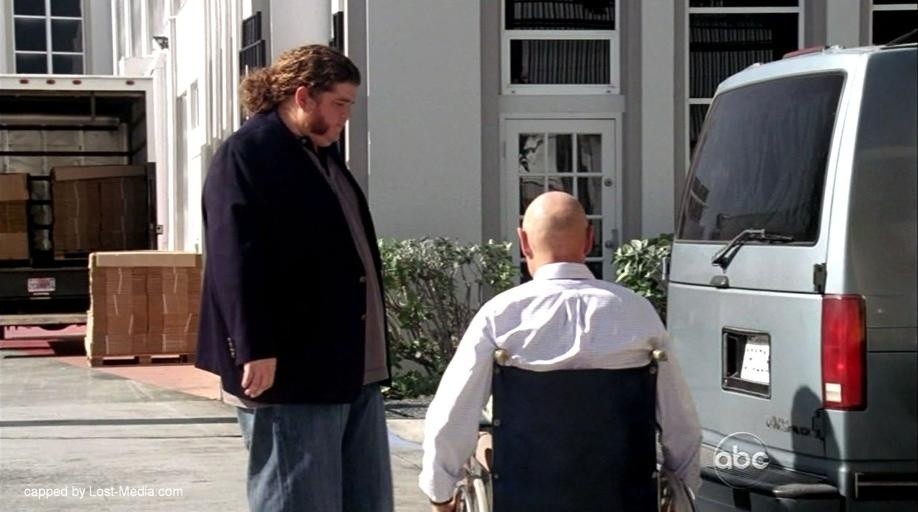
663 43 918 511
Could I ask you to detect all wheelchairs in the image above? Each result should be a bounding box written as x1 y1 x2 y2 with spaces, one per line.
451 346 696 512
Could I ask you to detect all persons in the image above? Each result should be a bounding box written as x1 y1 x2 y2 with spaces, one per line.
417 188 704 512
194 44 395 512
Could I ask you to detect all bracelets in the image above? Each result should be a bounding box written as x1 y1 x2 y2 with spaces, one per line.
428 495 454 506
